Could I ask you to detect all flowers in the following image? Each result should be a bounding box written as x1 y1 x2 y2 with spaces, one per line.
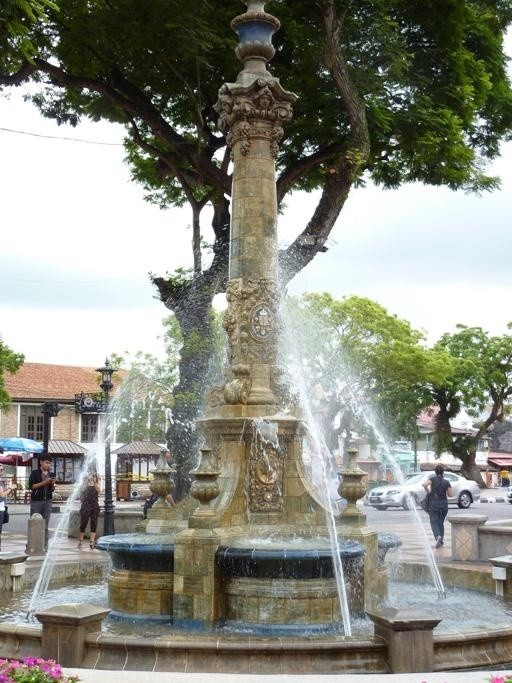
488 671 511 683
1 654 79 683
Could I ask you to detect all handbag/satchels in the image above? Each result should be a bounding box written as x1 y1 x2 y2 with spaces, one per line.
4 506 10 523
421 495 428 512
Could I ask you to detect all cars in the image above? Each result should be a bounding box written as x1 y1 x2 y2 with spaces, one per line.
368 471 480 510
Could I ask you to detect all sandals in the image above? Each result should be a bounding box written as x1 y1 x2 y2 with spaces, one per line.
78 540 94 549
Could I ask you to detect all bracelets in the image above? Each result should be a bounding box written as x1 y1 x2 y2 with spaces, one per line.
94 481 97 483
8 488 12 490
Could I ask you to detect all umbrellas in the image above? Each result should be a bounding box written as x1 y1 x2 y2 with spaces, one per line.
1 436 45 498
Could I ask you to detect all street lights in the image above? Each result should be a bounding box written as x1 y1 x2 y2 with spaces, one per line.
95 358 118 537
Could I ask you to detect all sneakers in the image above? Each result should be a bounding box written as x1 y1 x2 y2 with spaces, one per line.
435 535 444 548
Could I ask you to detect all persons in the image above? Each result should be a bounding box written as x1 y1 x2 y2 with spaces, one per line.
25 452 56 554
386 468 397 481
484 467 509 488
143 490 176 520
422 465 453 548
77 460 101 550
0 464 18 552
